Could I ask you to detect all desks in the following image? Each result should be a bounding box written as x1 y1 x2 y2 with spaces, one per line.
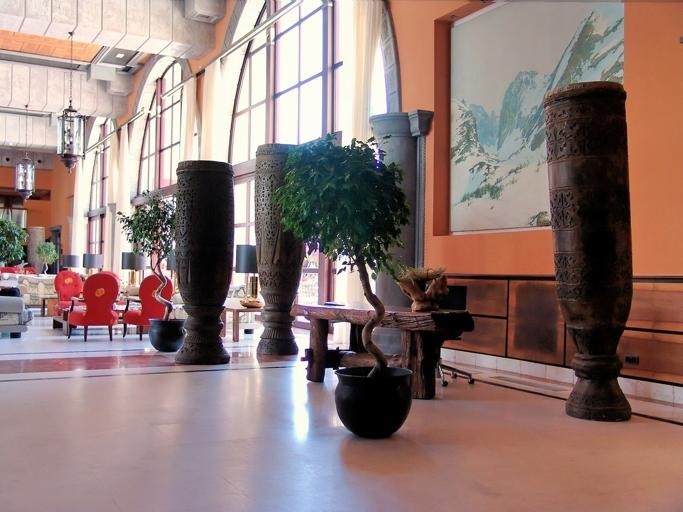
289 302 475 400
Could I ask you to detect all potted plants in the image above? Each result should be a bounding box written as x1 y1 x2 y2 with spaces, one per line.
113 190 187 353
270 131 414 440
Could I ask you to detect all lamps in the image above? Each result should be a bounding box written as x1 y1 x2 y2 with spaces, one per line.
62 244 265 308
15 104 36 203
56 31 84 175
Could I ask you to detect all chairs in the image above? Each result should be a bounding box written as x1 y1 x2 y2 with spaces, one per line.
434 284 475 387
0 296 34 339
54 270 174 342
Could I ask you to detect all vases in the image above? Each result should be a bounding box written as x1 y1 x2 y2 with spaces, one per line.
255 142 307 355
173 160 235 365
543 80 634 421
